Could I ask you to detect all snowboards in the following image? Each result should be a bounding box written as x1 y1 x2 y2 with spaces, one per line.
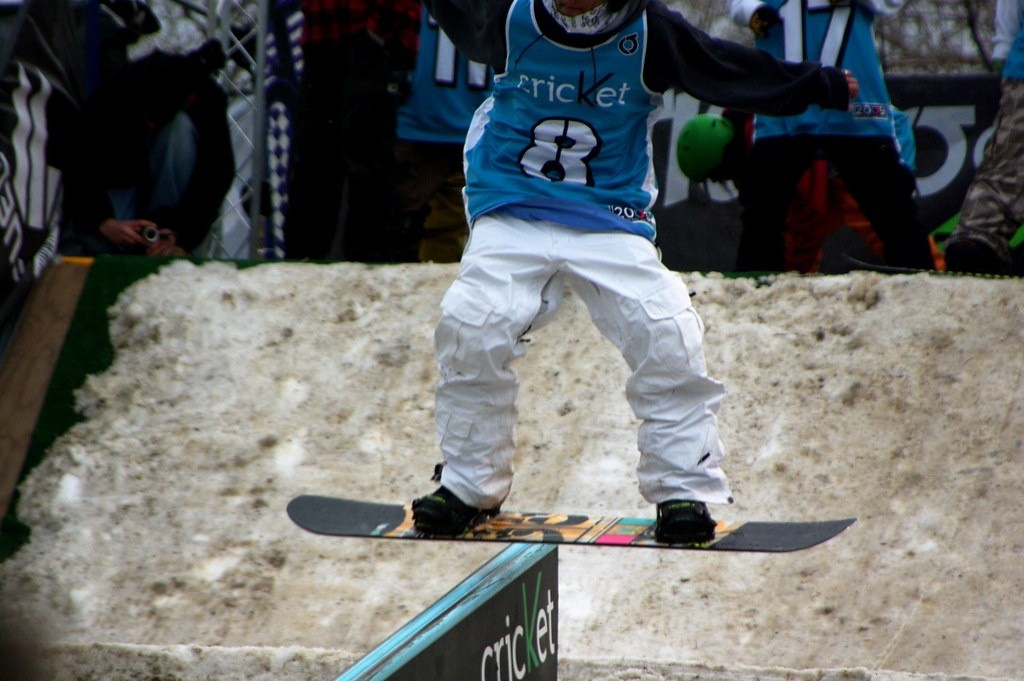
285 492 861 555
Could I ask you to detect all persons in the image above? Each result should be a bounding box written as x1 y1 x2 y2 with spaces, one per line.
372 0 500 265
717 0 937 277
940 0 1024 277
59 38 239 261
404 0 865 548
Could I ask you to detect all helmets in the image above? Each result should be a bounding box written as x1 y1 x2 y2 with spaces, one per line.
677 114 735 182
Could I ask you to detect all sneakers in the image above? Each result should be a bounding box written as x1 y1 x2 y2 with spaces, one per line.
412 486 501 535
654 500 717 548
